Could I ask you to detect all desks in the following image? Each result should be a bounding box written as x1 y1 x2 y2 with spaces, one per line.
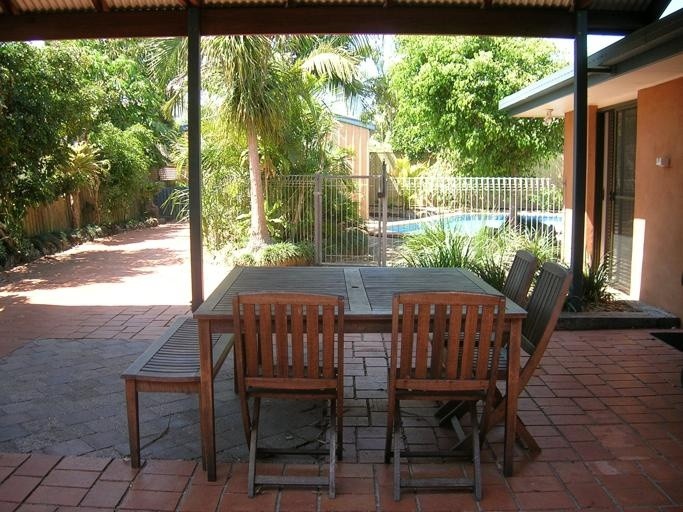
192 262 529 482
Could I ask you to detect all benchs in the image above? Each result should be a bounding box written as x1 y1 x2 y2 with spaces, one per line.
119 314 246 473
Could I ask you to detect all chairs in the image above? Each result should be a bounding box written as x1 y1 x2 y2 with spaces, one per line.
230 290 346 499
384 290 505 501
425 250 575 454
